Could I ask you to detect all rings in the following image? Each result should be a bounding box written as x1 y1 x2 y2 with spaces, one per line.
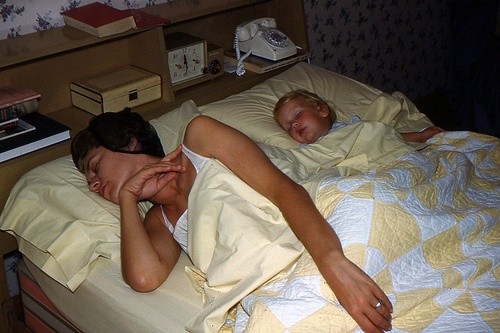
374 299 383 308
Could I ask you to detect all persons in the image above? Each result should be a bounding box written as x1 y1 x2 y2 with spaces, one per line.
72 107 393 333
273 90 443 147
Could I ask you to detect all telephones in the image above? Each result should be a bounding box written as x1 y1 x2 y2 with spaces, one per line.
233 17 298 62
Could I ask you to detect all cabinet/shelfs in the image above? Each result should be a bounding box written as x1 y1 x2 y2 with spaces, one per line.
0 0 311 214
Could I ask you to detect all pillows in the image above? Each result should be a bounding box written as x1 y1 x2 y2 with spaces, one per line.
198 61 382 149
0 100 197 293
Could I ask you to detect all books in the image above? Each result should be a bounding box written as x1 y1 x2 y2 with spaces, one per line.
60 2 169 38
224 48 311 74
0 88 71 164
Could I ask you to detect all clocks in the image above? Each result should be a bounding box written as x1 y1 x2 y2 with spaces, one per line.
166 31 209 87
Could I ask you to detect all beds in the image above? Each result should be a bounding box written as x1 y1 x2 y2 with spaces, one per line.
0 63 500 333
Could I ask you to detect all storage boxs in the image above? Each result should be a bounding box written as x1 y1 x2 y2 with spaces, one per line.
70 66 161 116
